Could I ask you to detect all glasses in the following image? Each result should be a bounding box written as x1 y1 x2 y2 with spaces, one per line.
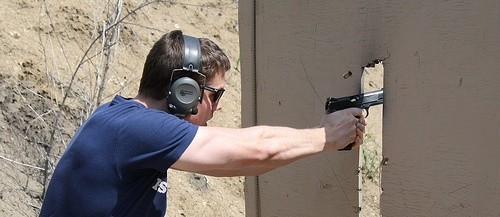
205 85 224 100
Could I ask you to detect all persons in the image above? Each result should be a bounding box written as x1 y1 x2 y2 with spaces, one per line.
38 30 367 217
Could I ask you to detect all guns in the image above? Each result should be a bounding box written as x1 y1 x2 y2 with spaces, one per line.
325 89 384 151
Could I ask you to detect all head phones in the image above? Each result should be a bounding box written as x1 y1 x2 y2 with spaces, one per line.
166 33 204 119
